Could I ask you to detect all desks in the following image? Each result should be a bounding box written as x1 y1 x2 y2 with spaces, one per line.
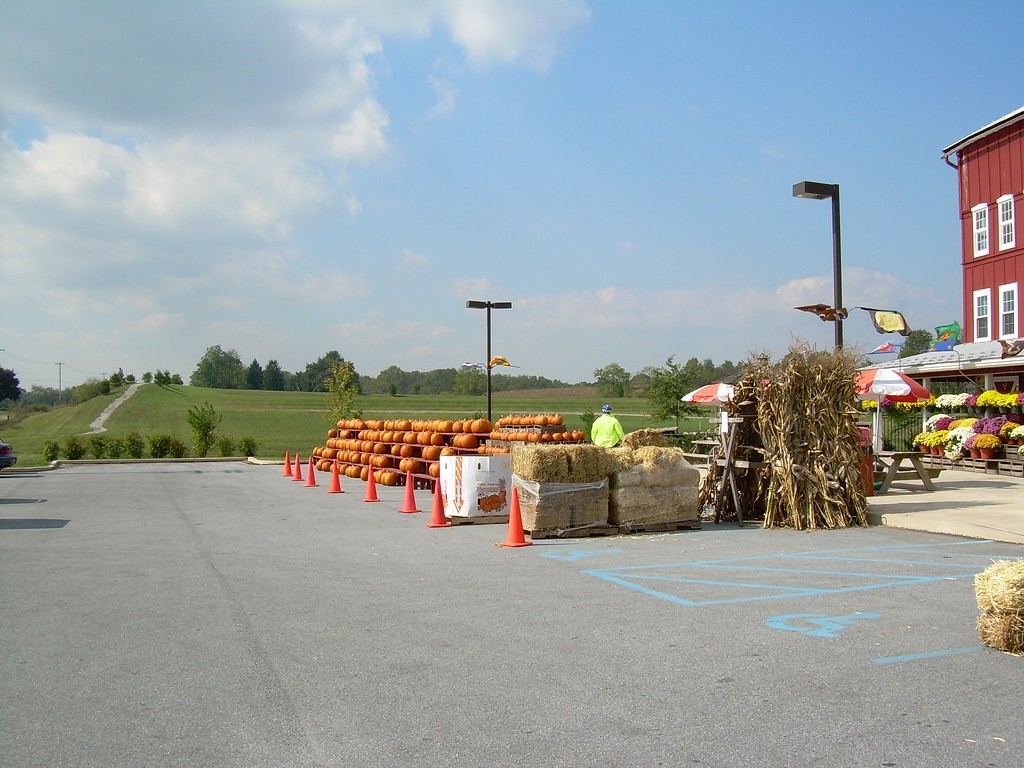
873 451 937 493
690 440 721 471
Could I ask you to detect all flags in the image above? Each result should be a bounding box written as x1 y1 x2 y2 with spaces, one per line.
925 320 961 351
859 306 913 335
997 336 1024 359
867 341 905 353
794 303 849 322
461 362 482 370
490 356 510 368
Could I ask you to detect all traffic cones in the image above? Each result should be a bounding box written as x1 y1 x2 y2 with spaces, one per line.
425 477 454 529
494 486 534 547
303 456 319 487
281 451 294 476
361 463 381 502
325 459 345 493
290 452 304 481
397 469 422 513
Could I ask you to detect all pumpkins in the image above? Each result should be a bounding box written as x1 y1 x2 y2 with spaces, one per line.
312 412 585 486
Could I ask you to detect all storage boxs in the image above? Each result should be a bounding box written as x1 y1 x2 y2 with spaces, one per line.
486 425 591 448
438 455 512 518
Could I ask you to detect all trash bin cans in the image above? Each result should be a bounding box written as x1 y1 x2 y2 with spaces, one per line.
855 425 876 498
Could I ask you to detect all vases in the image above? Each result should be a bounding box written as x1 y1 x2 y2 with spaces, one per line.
970 449 981 459
887 405 896 411
1010 405 1022 414
967 406 976 414
926 405 935 413
975 406 986 415
929 446 939 455
907 407 913 412
999 406 1011 414
1022 405 1024 414
869 407 881 413
919 444 931 454
914 407 922 412
1017 438 1024 445
997 435 1005 443
985 407 1000 416
951 407 960 413
960 405 968 413
961 447 971 458
938 445 946 456
1005 439 1017 445
940 404 951 414
979 448 996 459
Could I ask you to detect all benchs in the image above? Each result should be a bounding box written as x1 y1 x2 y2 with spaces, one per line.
873 471 888 494
682 453 718 470
885 466 947 493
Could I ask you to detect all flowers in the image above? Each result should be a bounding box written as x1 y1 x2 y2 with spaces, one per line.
990 393 1012 408
942 394 960 409
976 390 1001 406
861 400 878 409
956 392 973 406
1017 392 1024 405
1006 394 1020 406
879 399 895 406
964 392 981 406
895 392 936 412
935 393 952 407
909 413 1024 461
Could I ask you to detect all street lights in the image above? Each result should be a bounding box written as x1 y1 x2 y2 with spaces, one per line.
465 300 513 423
792 181 844 418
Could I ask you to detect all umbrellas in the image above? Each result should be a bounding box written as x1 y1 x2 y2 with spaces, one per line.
681 380 739 440
849 367 931 471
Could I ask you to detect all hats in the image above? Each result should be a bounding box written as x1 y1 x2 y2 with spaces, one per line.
601 405 612 414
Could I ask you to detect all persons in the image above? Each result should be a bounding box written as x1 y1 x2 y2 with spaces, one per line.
591 404 625 449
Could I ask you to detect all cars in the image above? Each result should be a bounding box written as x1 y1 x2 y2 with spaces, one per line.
0 438 18 471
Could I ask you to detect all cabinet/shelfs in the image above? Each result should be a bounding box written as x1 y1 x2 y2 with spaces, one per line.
312 424 491 494
